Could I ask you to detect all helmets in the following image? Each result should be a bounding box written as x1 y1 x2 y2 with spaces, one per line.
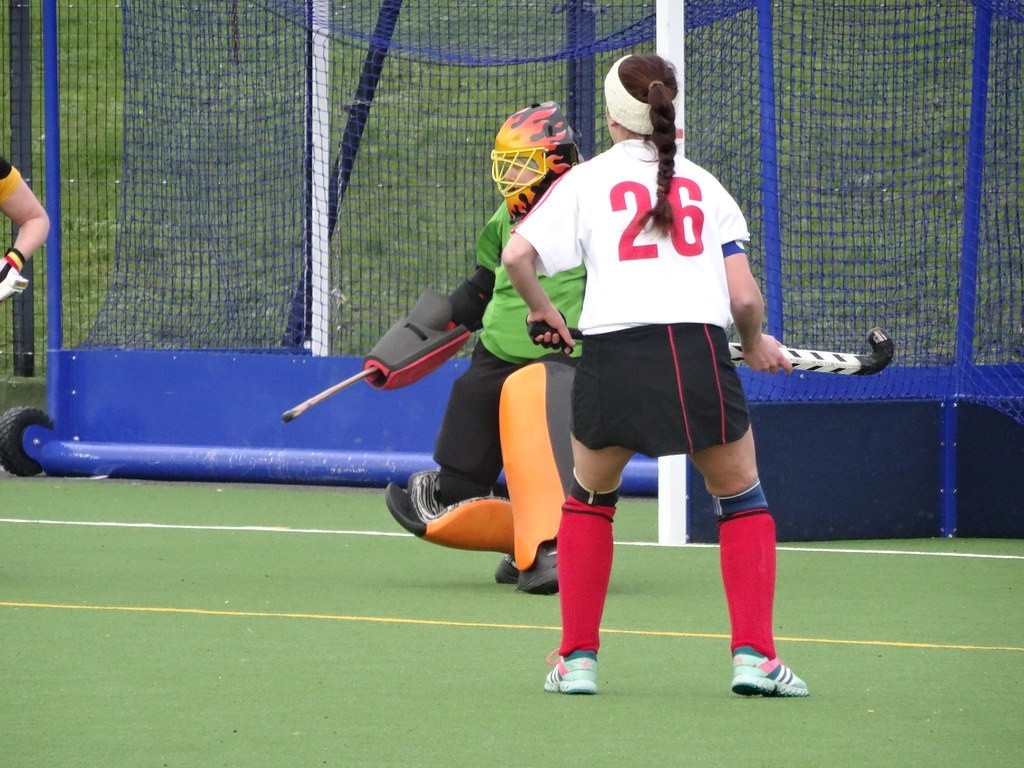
491 100 575 225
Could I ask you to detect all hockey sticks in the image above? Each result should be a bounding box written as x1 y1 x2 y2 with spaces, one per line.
526 322 895 375
282 365 380 422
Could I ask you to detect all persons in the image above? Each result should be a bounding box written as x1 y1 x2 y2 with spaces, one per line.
500 56 810 697
363 101 586 595
0 156 51 302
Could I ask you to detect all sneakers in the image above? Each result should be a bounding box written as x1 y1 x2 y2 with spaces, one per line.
544 647 598 694
494 544 563 595
730 646 808 696
